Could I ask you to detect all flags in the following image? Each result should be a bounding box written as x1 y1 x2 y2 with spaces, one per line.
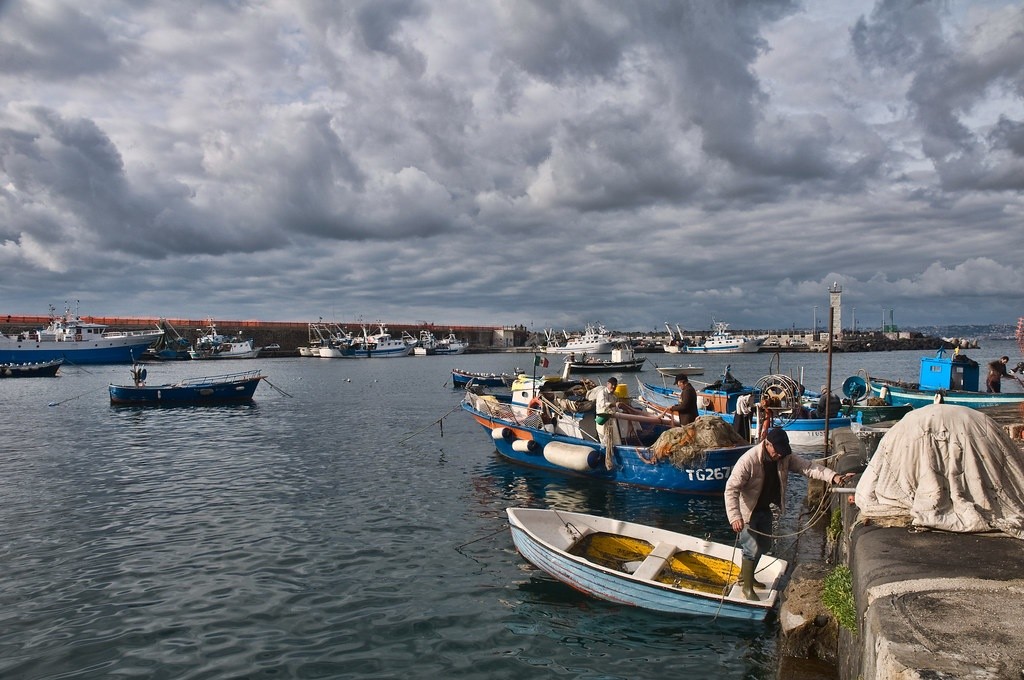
535 356 549 368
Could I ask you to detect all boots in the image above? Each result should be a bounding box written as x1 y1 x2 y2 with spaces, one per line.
738 557 766 601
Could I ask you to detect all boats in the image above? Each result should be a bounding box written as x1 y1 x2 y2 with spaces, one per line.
0 358 65 378
538 322 643 355
451 368 541 387
796 390 912 428
264 344 280 351
154 347 189 359
563 332 647 373
108 363 269 408
504 507 789 619
863 346 1024 409
186 318 263 360
634 374 857 444
662 313 771 353
296 314 469 358
656 364 703 377
460 377 756 494
0 300 166 365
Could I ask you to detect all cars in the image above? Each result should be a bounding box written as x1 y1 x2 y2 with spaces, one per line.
770 341 780 345
789 340 807 347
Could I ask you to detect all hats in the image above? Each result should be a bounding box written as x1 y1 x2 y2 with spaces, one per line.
607 377 617 385
821 385 826 392
673 374 687 385
767 428 792 455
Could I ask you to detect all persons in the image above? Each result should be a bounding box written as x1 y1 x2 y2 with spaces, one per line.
986 356 1018 393
817 385 840 419
724 428 855 601
667 374 699 427
596 377 622 445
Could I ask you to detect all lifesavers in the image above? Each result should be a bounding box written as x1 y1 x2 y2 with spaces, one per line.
528 397 544 417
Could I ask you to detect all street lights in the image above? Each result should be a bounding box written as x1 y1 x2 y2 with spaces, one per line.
882 308 885 336
